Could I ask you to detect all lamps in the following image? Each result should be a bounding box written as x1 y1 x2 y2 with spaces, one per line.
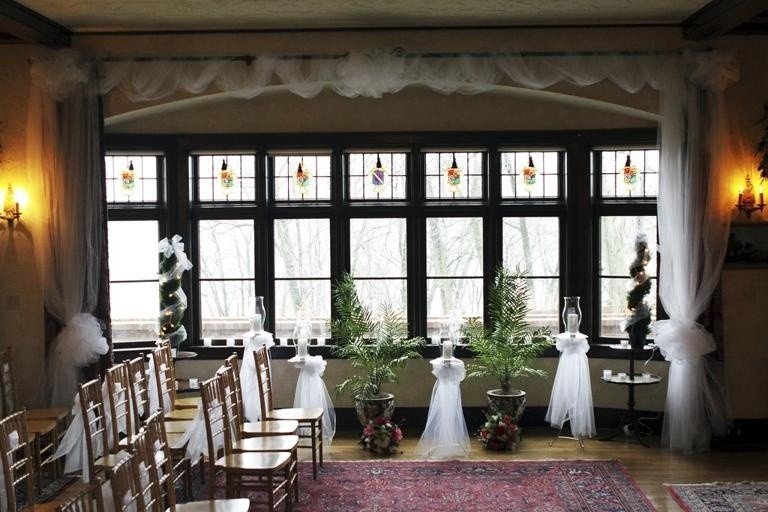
0 187 28 225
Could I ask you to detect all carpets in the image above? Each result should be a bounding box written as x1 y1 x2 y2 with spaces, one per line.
143 458 658 512
1 472 82 511
664 482 762 511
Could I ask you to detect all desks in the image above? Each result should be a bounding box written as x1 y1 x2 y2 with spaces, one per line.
601 372 664 447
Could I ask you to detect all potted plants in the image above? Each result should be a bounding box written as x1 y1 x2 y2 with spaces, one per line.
461 265 553 423
326 272 425 434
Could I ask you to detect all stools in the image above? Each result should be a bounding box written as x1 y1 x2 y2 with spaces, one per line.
4 419 57 495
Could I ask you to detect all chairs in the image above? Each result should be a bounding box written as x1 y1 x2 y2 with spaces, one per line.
151 345 212 482
152 341 204 411
59 481 107 511
197 373 292 512
128 426 160 512
105 360 193 500
126 351 207 490
225 352 298 492
144 408 251 512
77 376 164 498
252 344 325 480
218 367 300 506
146 351 199 406
111 455 144 512
0 349 71 476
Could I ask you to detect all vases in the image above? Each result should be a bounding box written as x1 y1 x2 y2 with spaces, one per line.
372 443 393 456
489 439 511 452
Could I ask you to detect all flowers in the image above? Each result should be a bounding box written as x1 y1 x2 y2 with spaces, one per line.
358 415 404 451
474 406 520 445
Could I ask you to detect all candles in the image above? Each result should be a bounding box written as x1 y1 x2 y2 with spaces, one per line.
759 193 765 205
739 194 746 207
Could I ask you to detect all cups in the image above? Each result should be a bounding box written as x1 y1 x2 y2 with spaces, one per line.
603 370 612 379
620 340 628 349
